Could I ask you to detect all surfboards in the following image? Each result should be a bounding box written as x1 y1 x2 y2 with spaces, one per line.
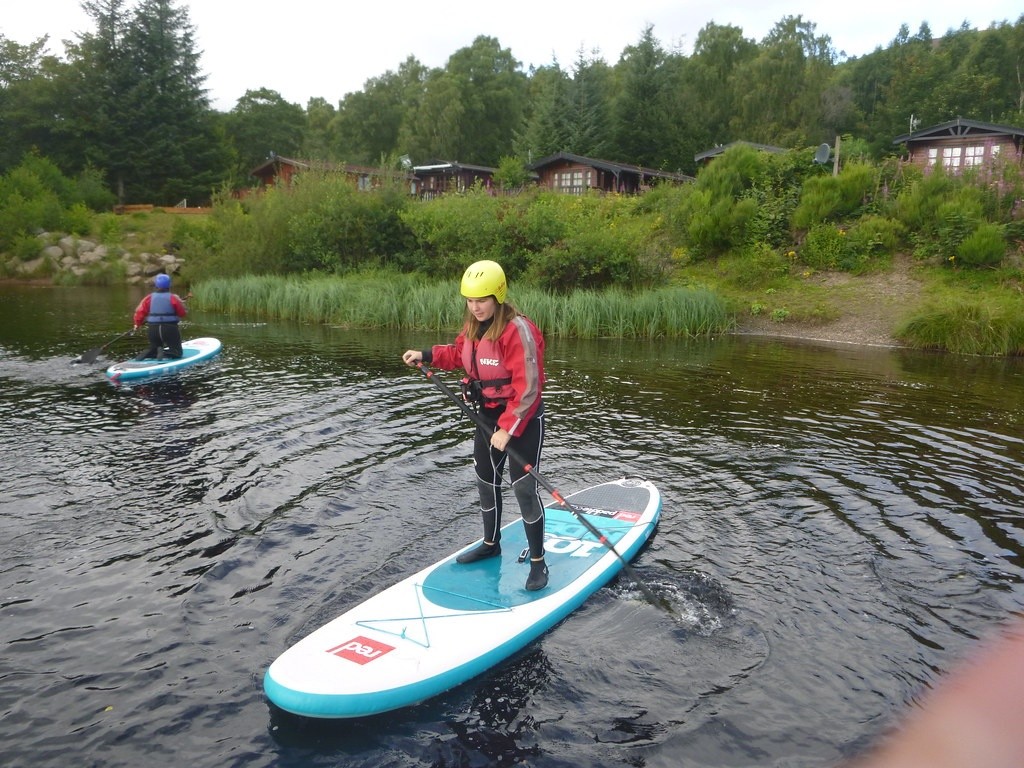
106 336 221 385
262 471 664 723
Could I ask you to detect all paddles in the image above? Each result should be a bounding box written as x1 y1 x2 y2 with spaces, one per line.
405 357 661 602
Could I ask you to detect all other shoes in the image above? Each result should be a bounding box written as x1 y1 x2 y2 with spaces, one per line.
136 349 149 360
526 559 549 590
157 347 163 360
456 542 501 563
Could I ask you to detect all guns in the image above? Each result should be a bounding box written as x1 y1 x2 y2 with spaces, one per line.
82 292 194 364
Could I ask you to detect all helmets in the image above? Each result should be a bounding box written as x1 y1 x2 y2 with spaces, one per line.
155 274 170 288
461 260 506 304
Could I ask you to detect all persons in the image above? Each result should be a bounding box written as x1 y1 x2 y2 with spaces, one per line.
402 259 551 591
133 273 190 361
